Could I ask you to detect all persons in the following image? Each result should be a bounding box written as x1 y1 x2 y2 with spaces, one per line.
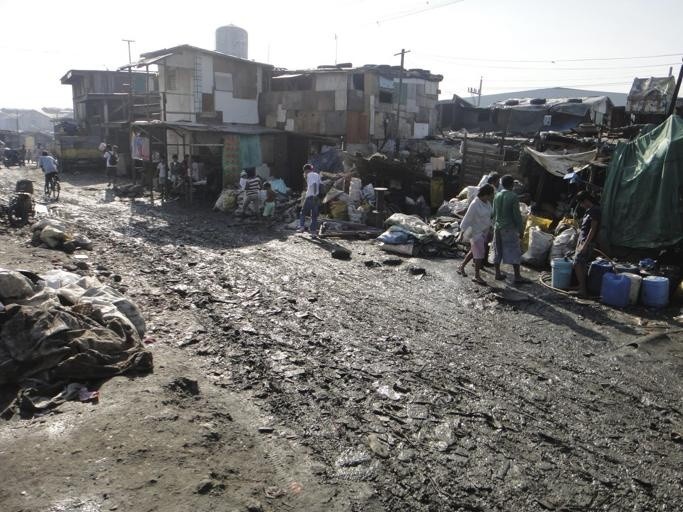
262 183 276 229
566 190 600 299
295 163 324 239
39 150 59 194
155 154 204 202
239 169 249 204
470 172 501 269
101 144 119 188
490 175 533 284
455 184 493 284
237 167 260 223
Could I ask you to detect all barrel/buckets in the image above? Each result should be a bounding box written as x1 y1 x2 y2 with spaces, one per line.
618 272 642 305
613 263 638 274
600 272 629 307
550 257 573 288
588 257 615 294
643 276 669 307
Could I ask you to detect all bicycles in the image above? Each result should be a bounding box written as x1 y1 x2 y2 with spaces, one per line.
44 172 62 199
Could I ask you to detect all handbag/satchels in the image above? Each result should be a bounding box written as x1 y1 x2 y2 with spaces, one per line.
488 243 500 264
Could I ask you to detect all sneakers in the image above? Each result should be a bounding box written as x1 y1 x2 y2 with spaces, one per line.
309 230 317 236
472 276 487 284
514 276 528 282
456 265 468 277
495 274 507 280
296 227 306 233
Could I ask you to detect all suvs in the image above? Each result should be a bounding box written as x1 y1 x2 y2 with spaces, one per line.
1 148 25 168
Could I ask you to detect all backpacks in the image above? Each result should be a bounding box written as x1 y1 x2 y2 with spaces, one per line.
107 151 118 166
